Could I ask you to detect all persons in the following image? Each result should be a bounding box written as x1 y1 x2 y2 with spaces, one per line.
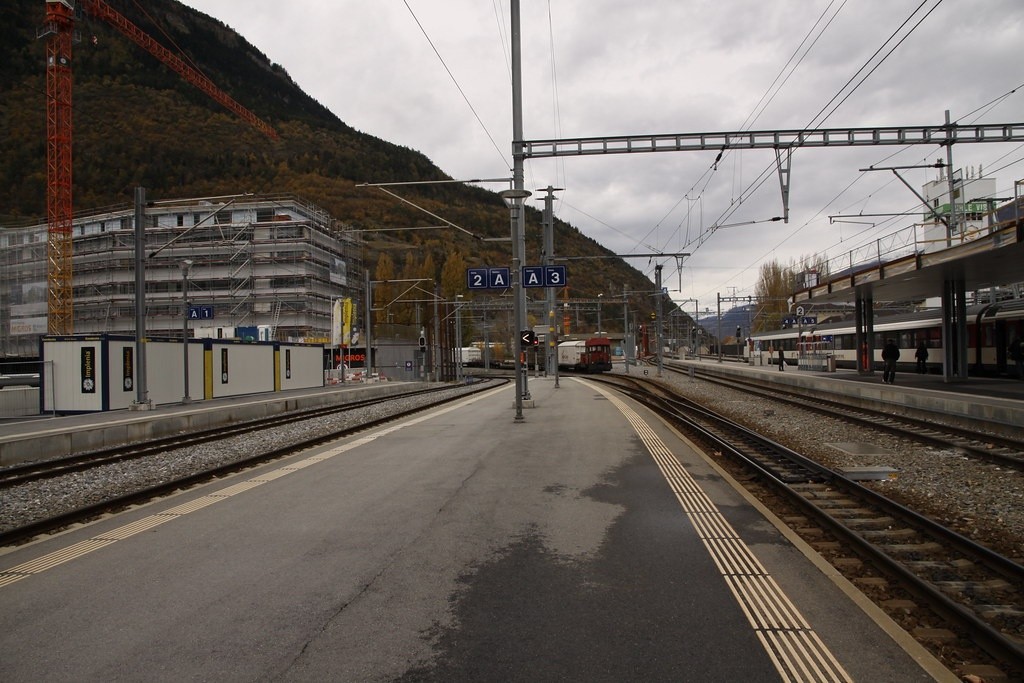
1010 336 1024 380
778 346 785 371
881 338 901 384
914 341 929 375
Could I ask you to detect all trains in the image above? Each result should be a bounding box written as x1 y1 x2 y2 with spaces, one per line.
451 333 613 374
740 296 1024 384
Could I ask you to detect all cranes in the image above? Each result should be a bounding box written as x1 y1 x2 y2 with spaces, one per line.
29 0 283 336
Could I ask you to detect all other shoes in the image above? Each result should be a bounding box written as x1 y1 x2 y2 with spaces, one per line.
881 378 891 384
780 369 784 371
